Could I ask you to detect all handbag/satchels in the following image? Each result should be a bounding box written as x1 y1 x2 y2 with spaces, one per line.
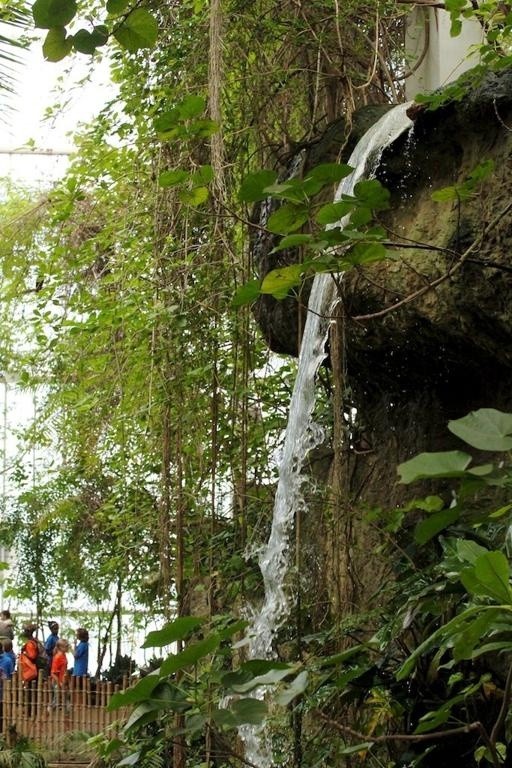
21 654 37 681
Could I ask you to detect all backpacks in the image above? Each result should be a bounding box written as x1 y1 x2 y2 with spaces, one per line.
29 638 51 672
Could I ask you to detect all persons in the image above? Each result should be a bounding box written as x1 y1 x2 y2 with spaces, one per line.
47 639 71 716
0 642 14 680
3 638 16 669
0 610 15 653
43 620 59 677
69 628 89 704
17 624 51 716
